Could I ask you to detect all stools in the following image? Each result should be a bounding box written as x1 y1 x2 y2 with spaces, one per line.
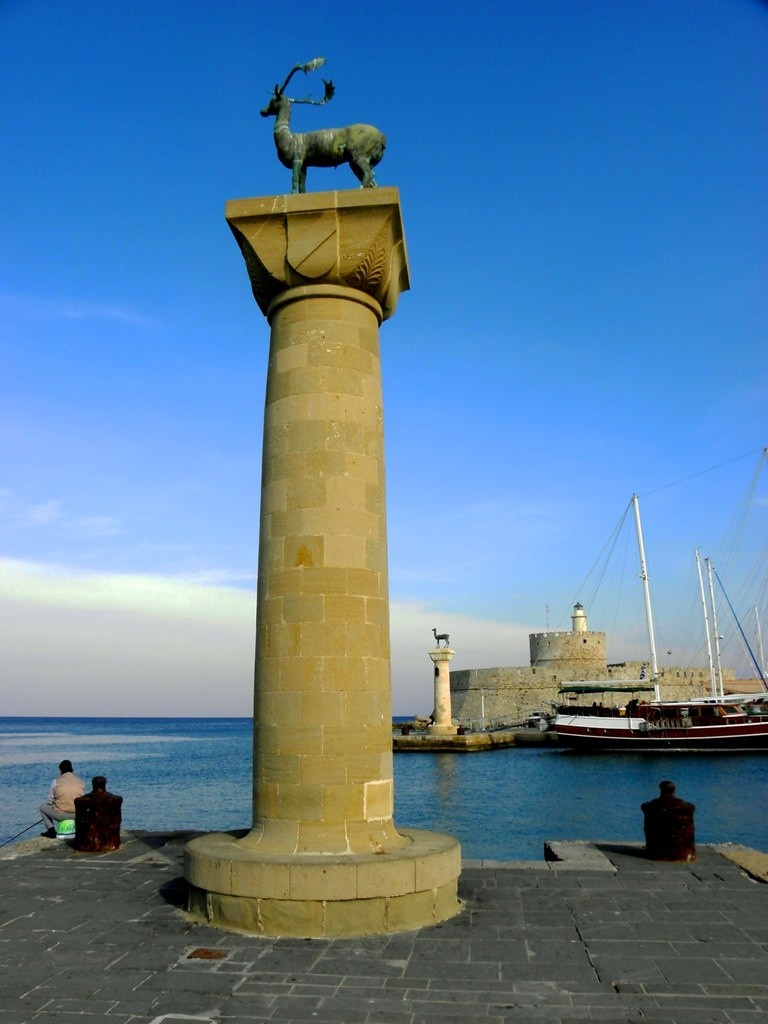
56 819 76 839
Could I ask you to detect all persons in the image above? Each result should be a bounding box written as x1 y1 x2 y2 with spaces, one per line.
39 760 85 839
593 702 597 714
597 702 603 715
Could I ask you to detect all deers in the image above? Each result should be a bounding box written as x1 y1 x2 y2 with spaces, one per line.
259 57 386 195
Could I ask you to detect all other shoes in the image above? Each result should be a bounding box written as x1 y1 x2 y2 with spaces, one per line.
40 827 57 838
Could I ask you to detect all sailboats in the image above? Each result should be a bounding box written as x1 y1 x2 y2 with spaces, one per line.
528 493 768 753
